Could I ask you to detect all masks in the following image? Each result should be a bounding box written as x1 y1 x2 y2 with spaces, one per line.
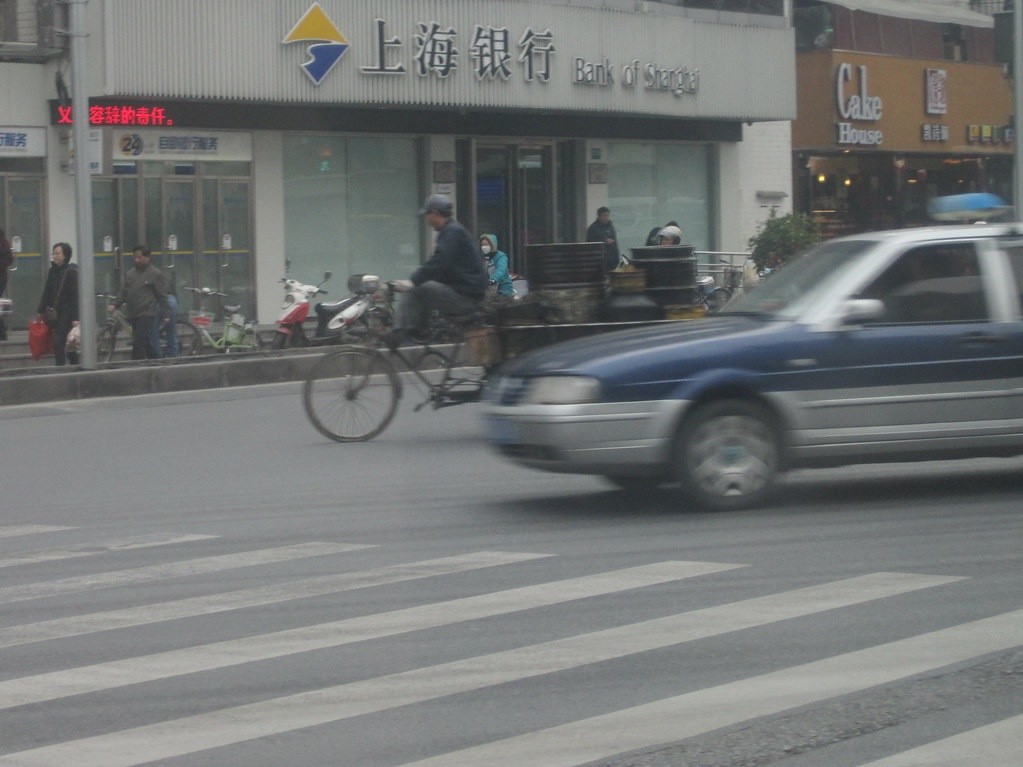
480 246 490 254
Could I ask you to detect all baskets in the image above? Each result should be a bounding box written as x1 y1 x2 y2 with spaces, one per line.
189 310 215 331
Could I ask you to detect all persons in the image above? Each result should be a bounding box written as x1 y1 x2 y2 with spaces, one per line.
35 243 80 366
645 221 681 246
107 245 181 360
0 228 13 340
477 234 513 299
587 207 620 270
384 195 488 346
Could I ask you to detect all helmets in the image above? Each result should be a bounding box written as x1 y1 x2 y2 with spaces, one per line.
656 226 682 245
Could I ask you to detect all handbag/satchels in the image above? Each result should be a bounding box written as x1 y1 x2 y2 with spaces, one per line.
29 314 53 360
64 322 82 357
45 306 57 319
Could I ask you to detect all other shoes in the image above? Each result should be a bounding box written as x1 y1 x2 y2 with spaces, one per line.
380 327 428 348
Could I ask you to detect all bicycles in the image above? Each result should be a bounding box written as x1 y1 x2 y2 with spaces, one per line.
299 281 576 446
184 288 264 358
694 259 749 313
93 288 202 370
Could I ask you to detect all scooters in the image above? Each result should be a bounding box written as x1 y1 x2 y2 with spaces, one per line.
270 257 392 351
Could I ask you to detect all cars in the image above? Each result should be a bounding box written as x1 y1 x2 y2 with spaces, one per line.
477 192 1023 512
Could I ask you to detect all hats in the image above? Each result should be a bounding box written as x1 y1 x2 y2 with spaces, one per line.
414 194 453 218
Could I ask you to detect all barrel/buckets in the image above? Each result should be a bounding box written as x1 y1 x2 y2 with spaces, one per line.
609 269 658 321
630 245 700 319
523 242 610 323
